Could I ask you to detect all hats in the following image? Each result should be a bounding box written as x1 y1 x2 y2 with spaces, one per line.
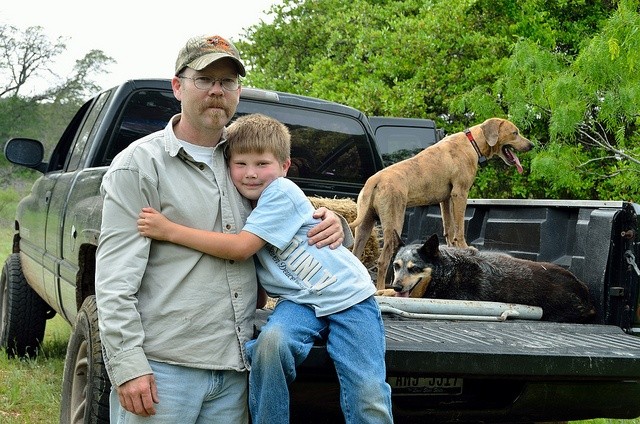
175 33 246 78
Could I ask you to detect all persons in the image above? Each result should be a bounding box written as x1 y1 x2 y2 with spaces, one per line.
95 34 345 423
137 113 394 423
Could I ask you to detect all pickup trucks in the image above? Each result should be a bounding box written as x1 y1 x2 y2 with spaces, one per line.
0 79 640 424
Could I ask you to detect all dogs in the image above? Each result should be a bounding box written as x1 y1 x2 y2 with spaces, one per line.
372 228 598 322
349 116 537 290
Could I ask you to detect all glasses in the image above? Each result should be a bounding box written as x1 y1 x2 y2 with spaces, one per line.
178 72 243 92
